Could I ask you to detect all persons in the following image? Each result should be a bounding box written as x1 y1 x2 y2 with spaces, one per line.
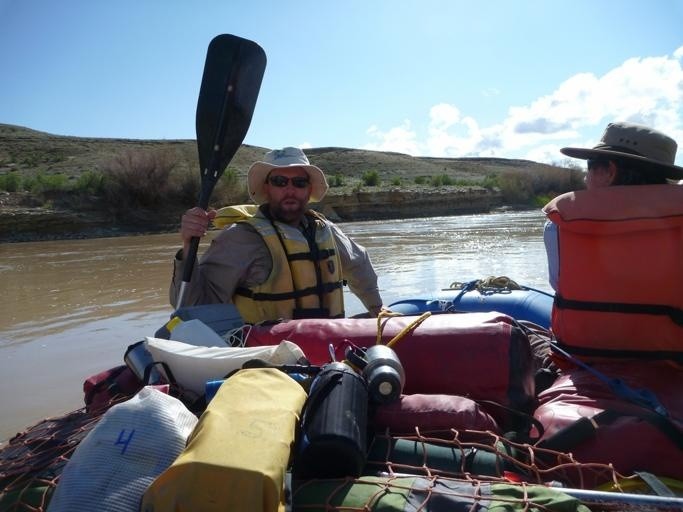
169 148 392 325
541 121 683 370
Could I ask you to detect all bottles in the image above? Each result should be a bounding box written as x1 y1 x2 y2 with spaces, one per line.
305 361 368 478
344 344 406 405
166 317 229 349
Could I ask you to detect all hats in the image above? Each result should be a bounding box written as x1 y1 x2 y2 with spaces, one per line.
247 146 329 205
560 121 683 181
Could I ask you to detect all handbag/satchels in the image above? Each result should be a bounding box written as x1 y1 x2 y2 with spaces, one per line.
46 383 199 511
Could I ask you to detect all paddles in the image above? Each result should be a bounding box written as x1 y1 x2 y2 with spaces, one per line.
176 33 266 309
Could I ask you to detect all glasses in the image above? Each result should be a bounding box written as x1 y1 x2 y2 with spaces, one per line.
265 176 311 188
